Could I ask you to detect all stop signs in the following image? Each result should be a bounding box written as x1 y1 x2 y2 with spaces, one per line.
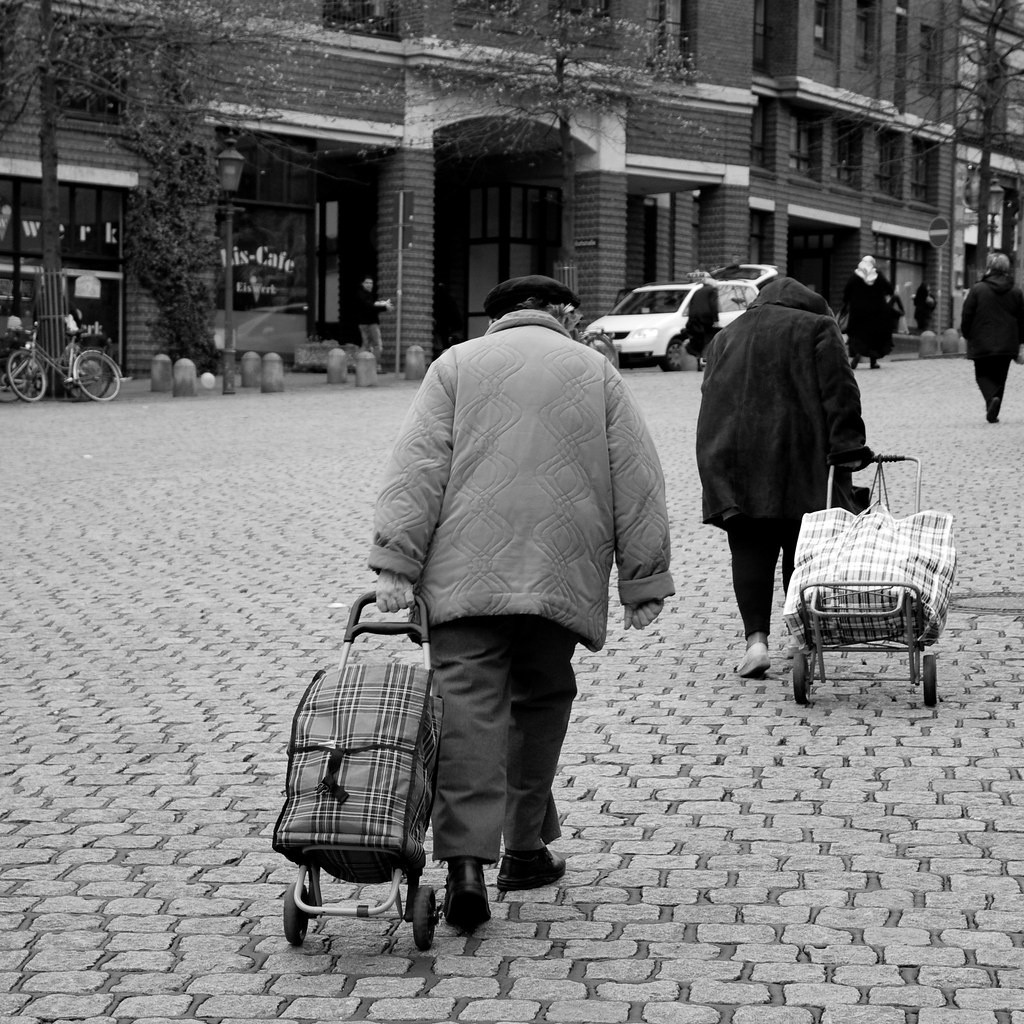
928 217 951 248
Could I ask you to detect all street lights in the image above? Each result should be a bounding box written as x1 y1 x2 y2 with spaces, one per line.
985 178 1005 254
214 131 246 394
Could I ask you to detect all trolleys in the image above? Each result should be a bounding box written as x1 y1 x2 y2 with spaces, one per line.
792 453 938 709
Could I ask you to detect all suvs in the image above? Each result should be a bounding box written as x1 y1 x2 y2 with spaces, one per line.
584 263 779 372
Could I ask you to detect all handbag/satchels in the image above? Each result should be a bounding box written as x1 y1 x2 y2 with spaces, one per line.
1014 344 1024 364
783 501 956 642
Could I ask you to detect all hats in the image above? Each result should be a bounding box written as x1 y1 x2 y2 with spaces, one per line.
484 275 580 311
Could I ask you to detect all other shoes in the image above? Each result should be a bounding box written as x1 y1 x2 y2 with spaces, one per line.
738 643 770 676
987 397 999 420
851 357 861 369
787 636 811 657
990 419 999 422
871 365 880 369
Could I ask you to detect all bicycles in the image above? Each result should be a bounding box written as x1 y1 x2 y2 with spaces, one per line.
0 344 114 402
575 328 620 372
6 317 123 402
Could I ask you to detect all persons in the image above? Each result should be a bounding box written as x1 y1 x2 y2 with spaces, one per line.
684 277 721 373
695 277 875 679
961 253 1024 423
356 275 395 373
365 274 676 927
912 281 937 335
840 255 895 369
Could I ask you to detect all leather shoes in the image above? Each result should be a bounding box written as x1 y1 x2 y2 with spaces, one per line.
497 847 566 891
443 864 491 923
